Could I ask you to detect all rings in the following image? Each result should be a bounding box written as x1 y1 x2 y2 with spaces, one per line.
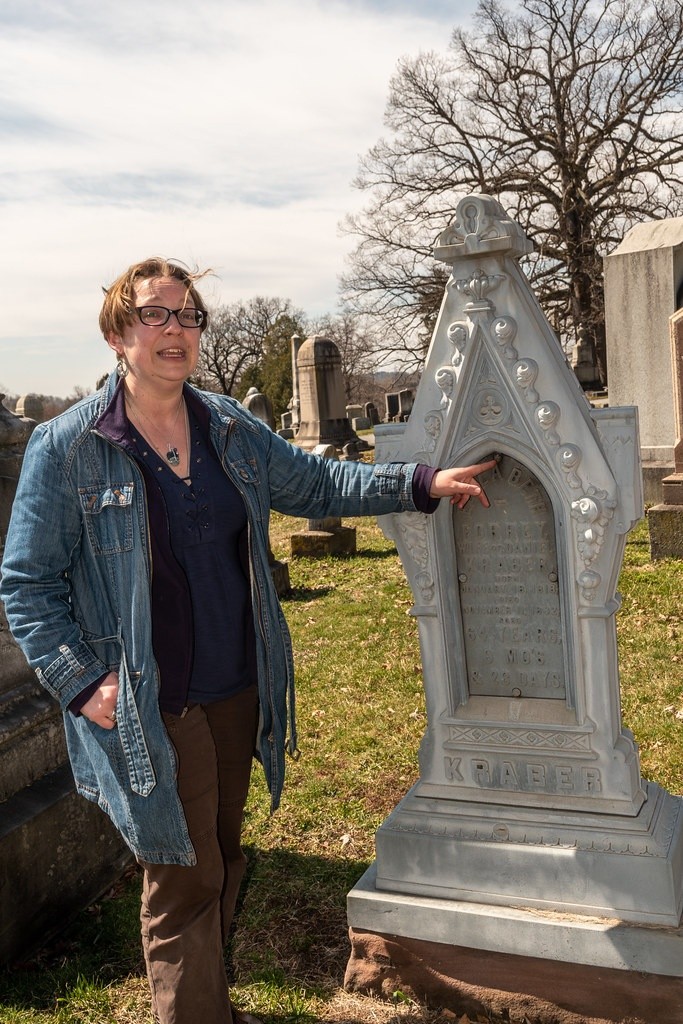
109 711 116 720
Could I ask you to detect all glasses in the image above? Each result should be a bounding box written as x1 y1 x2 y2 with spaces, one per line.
135 306 207 328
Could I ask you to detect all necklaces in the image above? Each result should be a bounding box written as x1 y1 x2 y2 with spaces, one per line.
125 391 190 476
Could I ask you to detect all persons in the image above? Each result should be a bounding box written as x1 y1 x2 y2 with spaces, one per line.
0 259 496 1024
